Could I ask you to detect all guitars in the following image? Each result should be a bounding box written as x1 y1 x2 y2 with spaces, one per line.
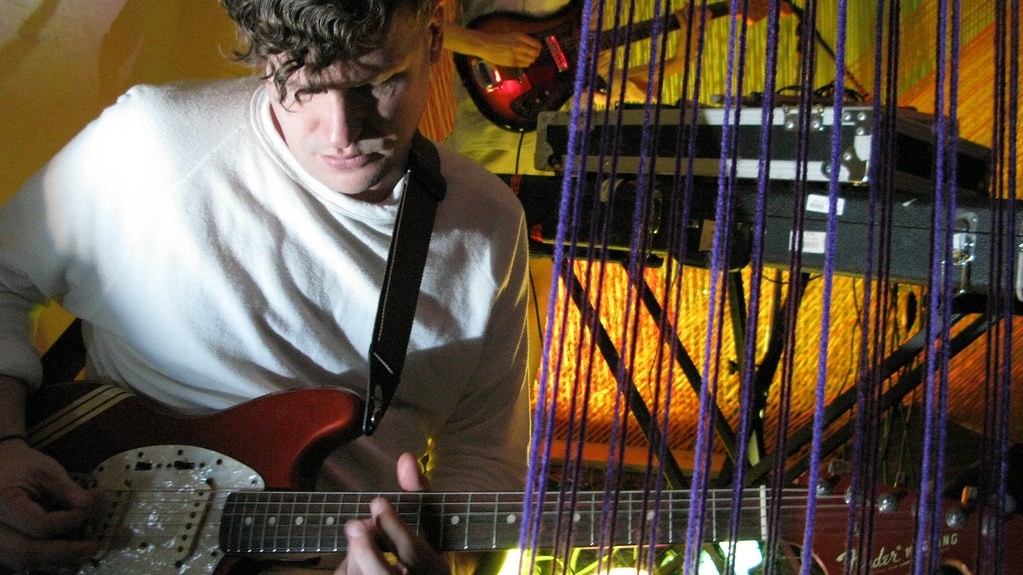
451 0 795 134
12 374 1023 575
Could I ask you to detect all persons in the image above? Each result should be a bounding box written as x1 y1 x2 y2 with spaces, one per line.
0 0 531 575
443 0 713 385
